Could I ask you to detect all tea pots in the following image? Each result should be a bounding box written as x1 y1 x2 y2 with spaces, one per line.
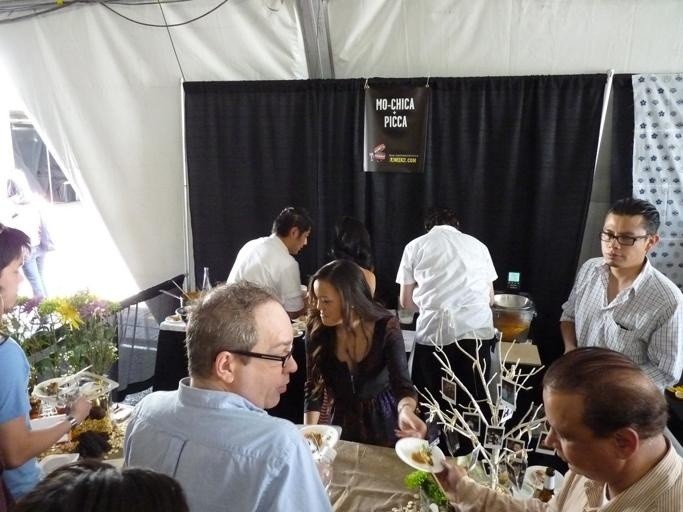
40 453 80 478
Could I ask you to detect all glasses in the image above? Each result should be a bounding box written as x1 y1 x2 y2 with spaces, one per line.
599 229 650 247
215 345 294 368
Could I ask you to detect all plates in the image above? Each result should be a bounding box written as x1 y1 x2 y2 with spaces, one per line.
297 424 336 456
395 437 446 473
290 316 309 341
526 465 564 490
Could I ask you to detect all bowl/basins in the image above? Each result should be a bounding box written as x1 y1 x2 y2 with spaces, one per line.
174 306 190 322
492 293 534 310
30 376 78 407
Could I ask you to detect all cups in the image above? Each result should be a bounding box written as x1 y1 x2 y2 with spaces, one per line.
510 484 537 501
398 296 413 325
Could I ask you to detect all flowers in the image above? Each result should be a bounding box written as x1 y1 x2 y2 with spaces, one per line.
0 291 123 394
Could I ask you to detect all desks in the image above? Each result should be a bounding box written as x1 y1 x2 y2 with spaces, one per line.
402 329 542 367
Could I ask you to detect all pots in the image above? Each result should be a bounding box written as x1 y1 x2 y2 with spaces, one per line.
157 288 202 306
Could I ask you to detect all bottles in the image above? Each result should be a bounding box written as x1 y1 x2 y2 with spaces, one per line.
201 267 214 294
538 467 556 503
